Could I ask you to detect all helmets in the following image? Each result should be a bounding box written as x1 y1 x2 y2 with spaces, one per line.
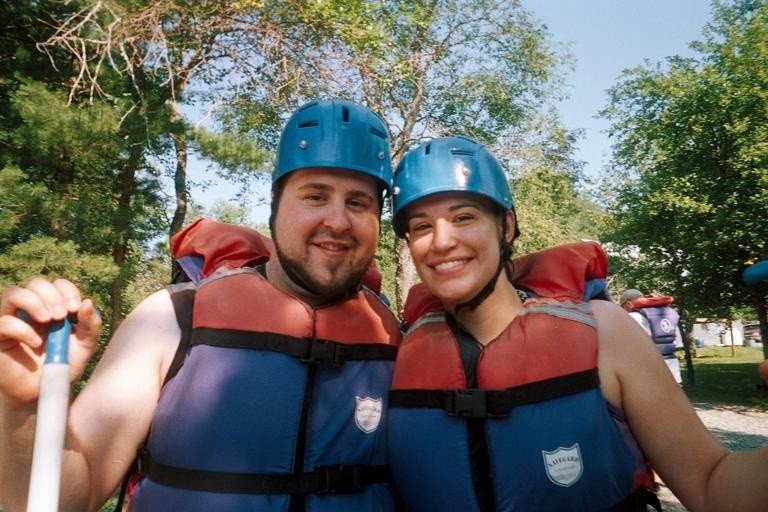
391 138 513 238
273 98 393 196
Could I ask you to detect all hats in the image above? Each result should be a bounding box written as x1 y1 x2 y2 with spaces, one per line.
620 288 643 306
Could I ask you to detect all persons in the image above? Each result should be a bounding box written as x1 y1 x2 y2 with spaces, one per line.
0 97 402 512
384 134 767 512
618 289 685 495
